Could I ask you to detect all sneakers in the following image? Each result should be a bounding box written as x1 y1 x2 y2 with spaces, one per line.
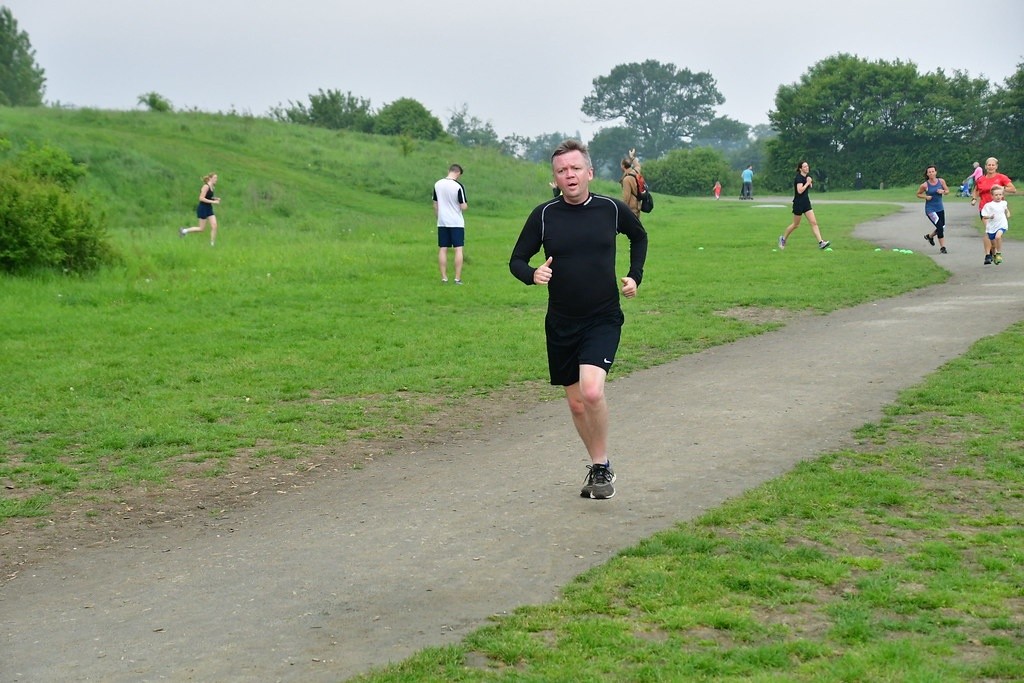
580 465 593 499
940 247 947 253
984 255 991 264
924 234 935 246
780 236 786 248
820 241 830 248
994 253 1002 265
591 461 615 499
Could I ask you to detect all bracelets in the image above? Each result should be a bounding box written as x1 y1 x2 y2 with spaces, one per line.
972 198 977 201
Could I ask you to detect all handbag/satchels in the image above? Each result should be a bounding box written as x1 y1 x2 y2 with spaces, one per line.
639 192 654 213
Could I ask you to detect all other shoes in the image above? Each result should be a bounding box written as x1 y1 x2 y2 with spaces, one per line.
990 250 993 261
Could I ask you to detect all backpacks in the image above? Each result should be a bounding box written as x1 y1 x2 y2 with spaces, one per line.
625 169 648 200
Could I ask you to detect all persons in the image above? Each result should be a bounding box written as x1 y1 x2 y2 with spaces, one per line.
970 157 1016 265
963 161 983 183
778 162 830 249
981 184 1011 265
713 181 721 200
432 164 468 284
548 181 563 197
179 172 221 246
619 148 642 219
507 141 648 499
741 165 753 200
916 166 950 253
962 180 971 197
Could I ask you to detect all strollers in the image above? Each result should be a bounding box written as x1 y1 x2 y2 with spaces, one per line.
739 183 750 200
956 178 974 197
